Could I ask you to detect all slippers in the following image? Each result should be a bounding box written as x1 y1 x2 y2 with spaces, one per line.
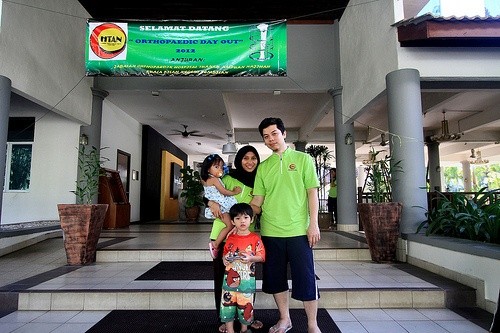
269 325 292 333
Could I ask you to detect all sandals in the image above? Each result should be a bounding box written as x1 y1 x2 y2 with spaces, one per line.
219 324 227 333
251 320 263 329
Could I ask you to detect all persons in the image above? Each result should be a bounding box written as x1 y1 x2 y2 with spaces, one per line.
328 168 337 228
201 145 263 333
219 203 266 333
227 117 321 333
201 154 242 259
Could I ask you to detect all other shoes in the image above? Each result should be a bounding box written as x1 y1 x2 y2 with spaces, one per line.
208 242 219 259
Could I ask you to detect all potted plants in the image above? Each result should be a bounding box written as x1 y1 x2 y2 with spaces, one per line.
307 145 335 230
179 166 203 224
57 144 110 266
357 150 403 264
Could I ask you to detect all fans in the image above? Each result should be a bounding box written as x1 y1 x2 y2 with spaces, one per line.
167 124 204 137
369 134 391 147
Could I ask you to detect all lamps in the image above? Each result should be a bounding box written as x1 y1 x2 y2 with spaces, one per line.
430 112 460 142
